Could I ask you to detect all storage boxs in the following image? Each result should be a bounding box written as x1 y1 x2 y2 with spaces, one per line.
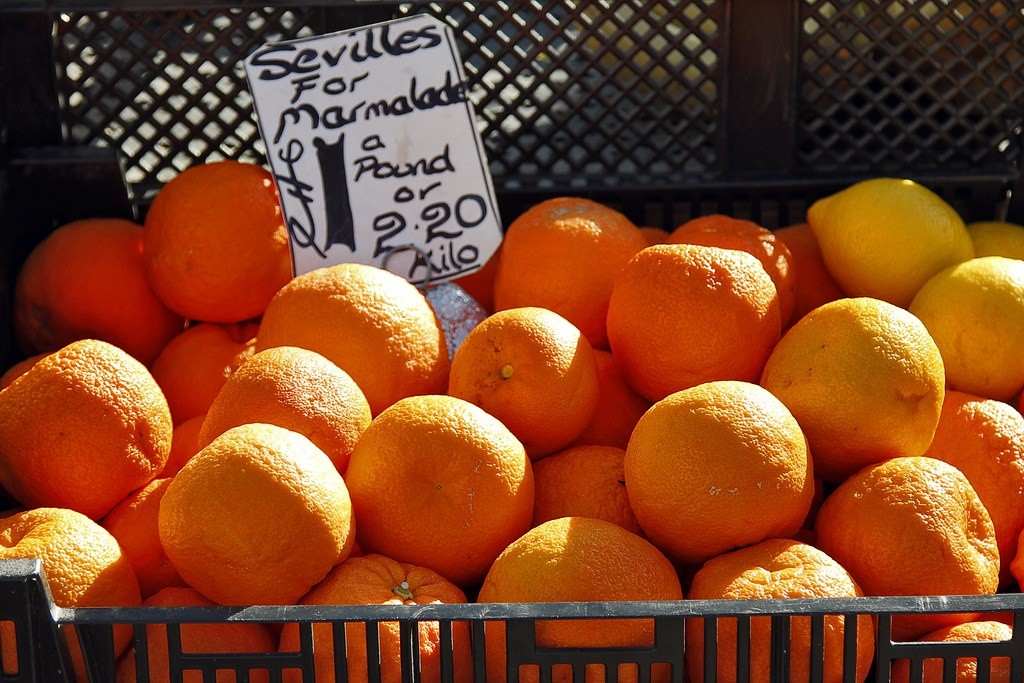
0 144 1022 683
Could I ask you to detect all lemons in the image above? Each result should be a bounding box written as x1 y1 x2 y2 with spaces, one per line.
804 176 977 310
968 221 1024 259
909 256 1023 400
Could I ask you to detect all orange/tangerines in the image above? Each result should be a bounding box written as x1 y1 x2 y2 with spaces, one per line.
920 390 1024 584
0 158 878 683
761 294 947 470
891 620 1013 682
814 457 999 638
